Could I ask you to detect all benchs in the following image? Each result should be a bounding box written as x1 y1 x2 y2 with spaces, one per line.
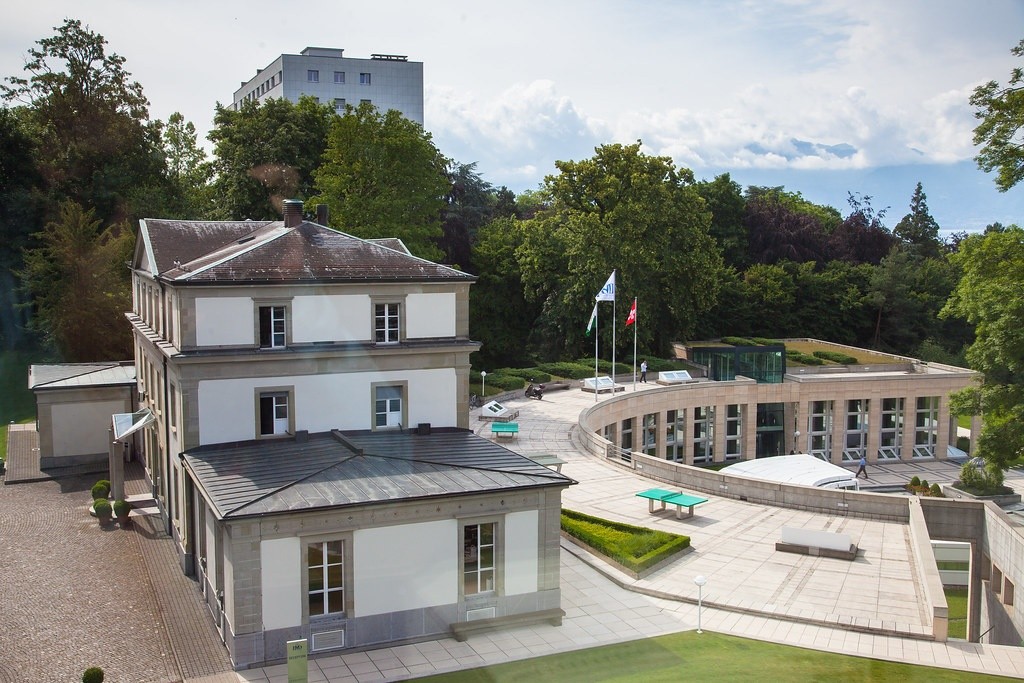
450 607 566 643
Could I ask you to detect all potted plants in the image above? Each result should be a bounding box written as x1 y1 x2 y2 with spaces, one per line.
113 500 132 526
91 480 112 525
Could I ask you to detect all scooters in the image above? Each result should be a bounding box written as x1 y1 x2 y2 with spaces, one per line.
524 379 545 400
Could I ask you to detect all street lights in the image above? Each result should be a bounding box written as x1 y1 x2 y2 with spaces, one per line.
481 371 487 397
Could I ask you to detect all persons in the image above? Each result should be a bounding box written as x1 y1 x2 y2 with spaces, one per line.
640 361 647 383
790 450 794 455
798 451 802 454
856 455 868 478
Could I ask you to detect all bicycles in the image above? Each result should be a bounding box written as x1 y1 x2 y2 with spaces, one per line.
469 393 481 410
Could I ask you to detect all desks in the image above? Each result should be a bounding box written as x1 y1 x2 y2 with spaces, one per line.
526 451 568 473
491 421 519 442
636 486 707 519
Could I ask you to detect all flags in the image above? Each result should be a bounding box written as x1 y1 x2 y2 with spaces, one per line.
626 300 635 326
595 271 615 301
586 304 597 337
